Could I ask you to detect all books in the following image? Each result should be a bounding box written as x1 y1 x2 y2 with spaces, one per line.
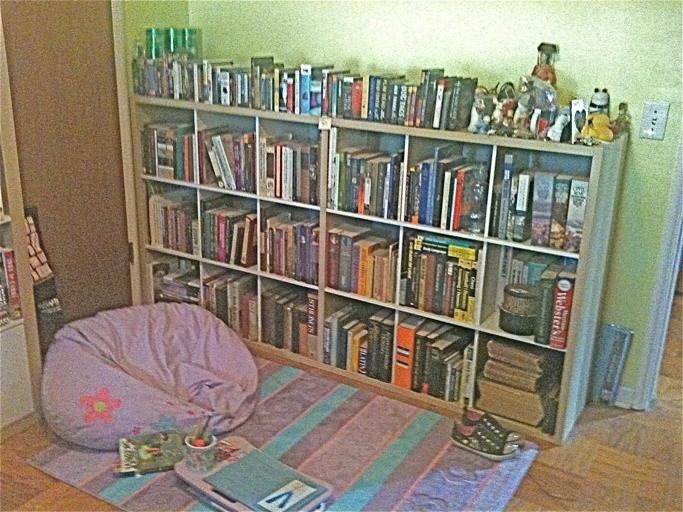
118 428 185 478
0 188 24 331
148 182 581 349
132 26 478 132
137 118 592 255
202 448 328 512
148 255 562 437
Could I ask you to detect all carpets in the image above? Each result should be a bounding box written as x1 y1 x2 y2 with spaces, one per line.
27 354 539 512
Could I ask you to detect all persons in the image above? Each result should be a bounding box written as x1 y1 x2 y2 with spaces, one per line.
466 42 633 147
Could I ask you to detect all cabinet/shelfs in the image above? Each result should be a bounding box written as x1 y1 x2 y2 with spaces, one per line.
1 21 43 444
135 94 628 444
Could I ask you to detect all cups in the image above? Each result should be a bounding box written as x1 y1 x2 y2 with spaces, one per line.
185 435 217 473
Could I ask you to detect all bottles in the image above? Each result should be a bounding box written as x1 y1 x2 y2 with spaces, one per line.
145 27 196 59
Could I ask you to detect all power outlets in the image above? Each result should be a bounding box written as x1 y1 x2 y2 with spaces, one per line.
639 102 670 140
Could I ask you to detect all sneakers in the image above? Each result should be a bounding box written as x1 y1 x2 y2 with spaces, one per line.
449 420 519 461
462 406 522 446
